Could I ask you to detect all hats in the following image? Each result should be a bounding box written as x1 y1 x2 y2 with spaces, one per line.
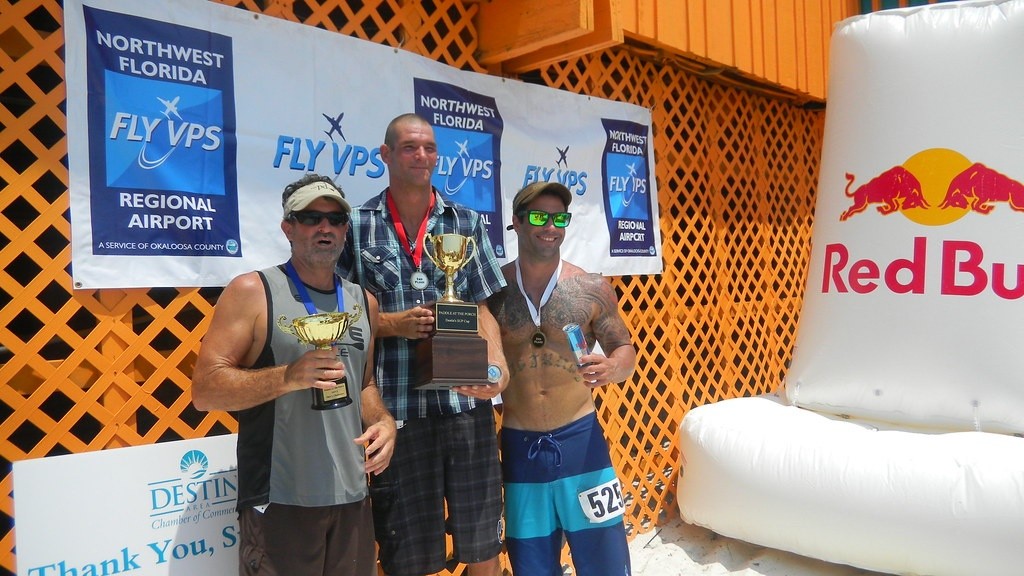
283 181 352 216
513 182 572 212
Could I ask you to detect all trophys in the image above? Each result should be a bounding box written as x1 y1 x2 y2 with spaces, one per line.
274 304 363 411
420 233 480 336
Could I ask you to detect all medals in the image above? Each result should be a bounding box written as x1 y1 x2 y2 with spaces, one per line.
530 326 546 347
409 271 430 291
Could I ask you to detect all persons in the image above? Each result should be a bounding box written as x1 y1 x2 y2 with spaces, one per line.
486 181 638 576
334 113 510 575
189 172 399 575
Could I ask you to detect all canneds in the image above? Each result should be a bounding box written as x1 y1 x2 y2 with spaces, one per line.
562 322 592 367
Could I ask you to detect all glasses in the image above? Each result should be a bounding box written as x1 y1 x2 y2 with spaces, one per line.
516 209 573 228
290 210 350 226
